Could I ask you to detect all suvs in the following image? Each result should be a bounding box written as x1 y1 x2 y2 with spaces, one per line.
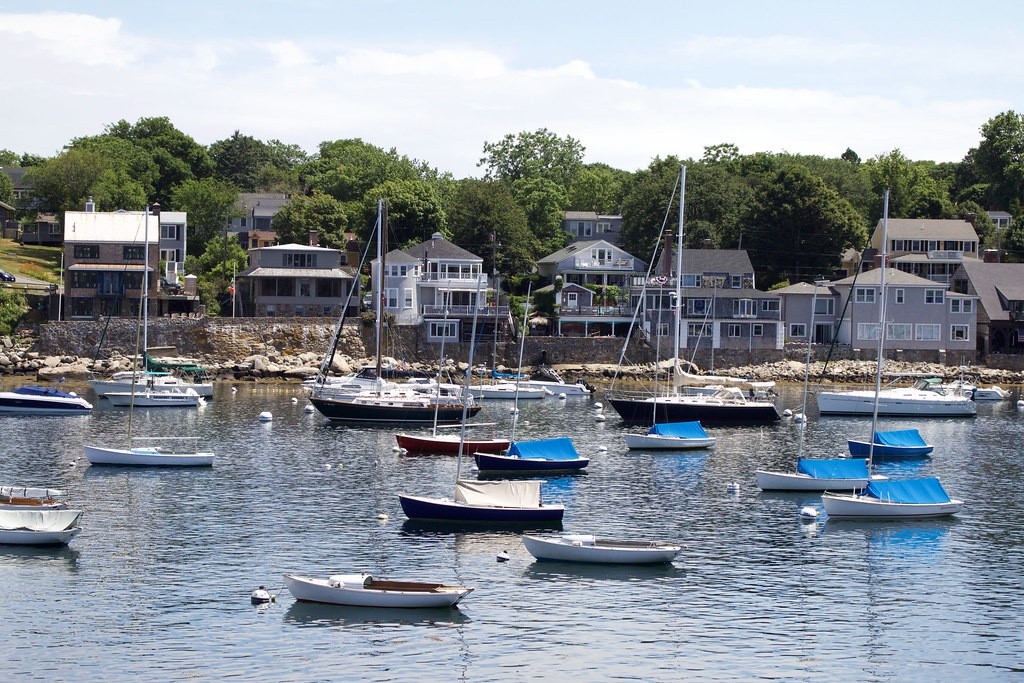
160 278 184 295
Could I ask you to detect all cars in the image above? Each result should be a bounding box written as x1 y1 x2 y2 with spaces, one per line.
0 268 16 282
362 292 373 310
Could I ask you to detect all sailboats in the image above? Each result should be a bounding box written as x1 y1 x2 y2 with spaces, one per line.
623 282 718 454
394 278 508 455
466 276 553 400
804 183 977 417
84 271 215 468
97 204 214 407
398 274 565 533
604 163 780 424
301 197 482 426
753 283 891 492
474 279 590 473
821 283 964 521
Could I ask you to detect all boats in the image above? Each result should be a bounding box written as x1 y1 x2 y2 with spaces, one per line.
0 375 94 413
281 572 474 607
848 429 933 458
0 487 84 545
932 380 1013 401
499 376 595 396
521 534 681 563
